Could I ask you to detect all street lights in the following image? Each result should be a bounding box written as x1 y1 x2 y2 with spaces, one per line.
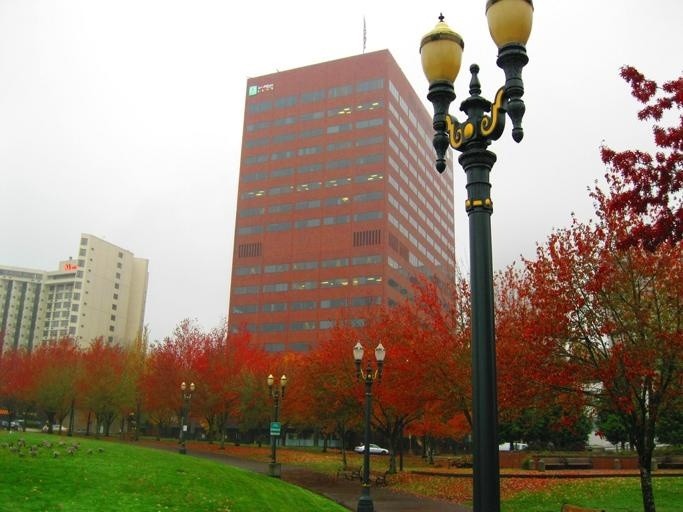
267 374 287 463
352 340 386 512
417 0 535 509
179 382 195 448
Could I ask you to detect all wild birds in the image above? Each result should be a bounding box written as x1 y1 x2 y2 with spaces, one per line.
0 438 104 457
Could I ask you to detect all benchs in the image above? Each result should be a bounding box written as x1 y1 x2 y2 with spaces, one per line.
535 456 594 469
337 464 387 488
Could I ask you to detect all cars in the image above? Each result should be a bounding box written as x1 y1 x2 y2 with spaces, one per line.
42 424 71 432
353 443 389 456
498 439 528 452
7 422 22 431
